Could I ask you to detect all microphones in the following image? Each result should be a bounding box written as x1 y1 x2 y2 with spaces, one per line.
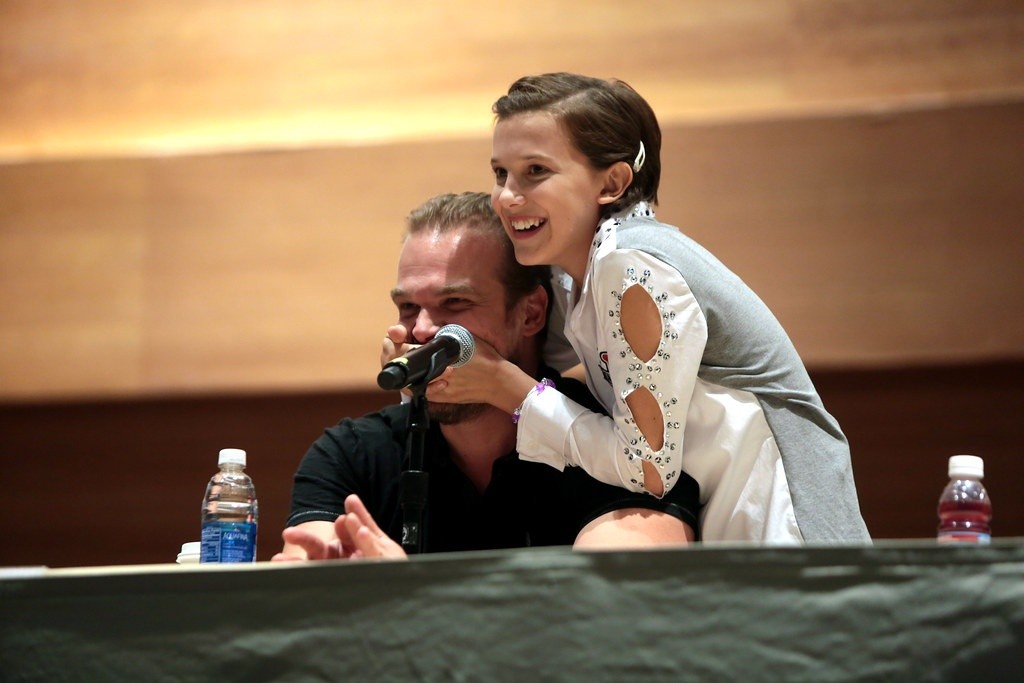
376 325 476 393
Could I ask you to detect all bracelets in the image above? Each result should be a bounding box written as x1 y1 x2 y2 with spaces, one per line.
507 376 556 428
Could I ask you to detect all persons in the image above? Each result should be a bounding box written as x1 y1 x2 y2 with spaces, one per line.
386 71 872 545
268 193 708 561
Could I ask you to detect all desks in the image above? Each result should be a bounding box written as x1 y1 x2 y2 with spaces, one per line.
0 535 1024 683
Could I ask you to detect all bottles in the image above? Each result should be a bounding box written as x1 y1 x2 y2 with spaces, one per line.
937 454 992 544
201 447 258 566
